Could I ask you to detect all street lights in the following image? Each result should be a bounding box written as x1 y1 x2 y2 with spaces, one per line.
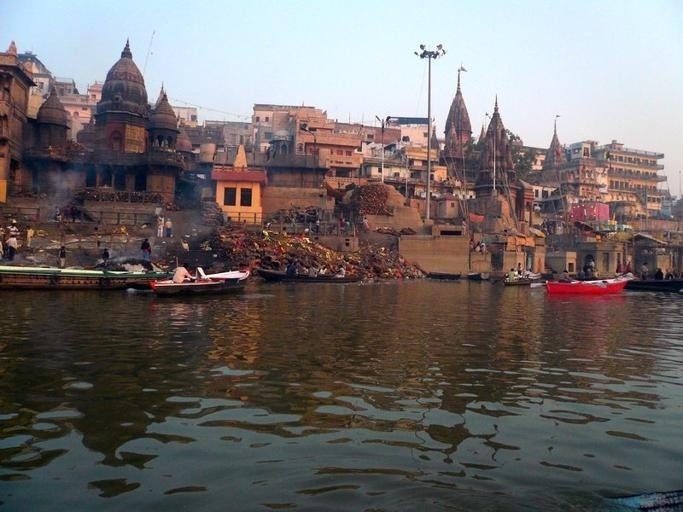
376 115 392 184
415 43 447 218
300 127 317 188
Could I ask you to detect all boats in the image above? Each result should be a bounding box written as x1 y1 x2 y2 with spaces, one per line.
0 265 179 289
428 271 682 294
147 265 250 292
257 258 361 283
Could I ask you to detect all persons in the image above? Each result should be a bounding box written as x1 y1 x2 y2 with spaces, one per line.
640 263 683 280
96 234 101 247
156 213 172 238
559 270 572 283
242 220 247 235
102 249 108 263
181 239 189 257
141 239 151 261
360 217 369 234
58 246 66 268
615 260 633 273
577 258 600 280
262 222 272 242
284 260 345 279
172 262 192 284
54 206 60 220
0 219 20 261
509 262 521 282
27 226 33 248
